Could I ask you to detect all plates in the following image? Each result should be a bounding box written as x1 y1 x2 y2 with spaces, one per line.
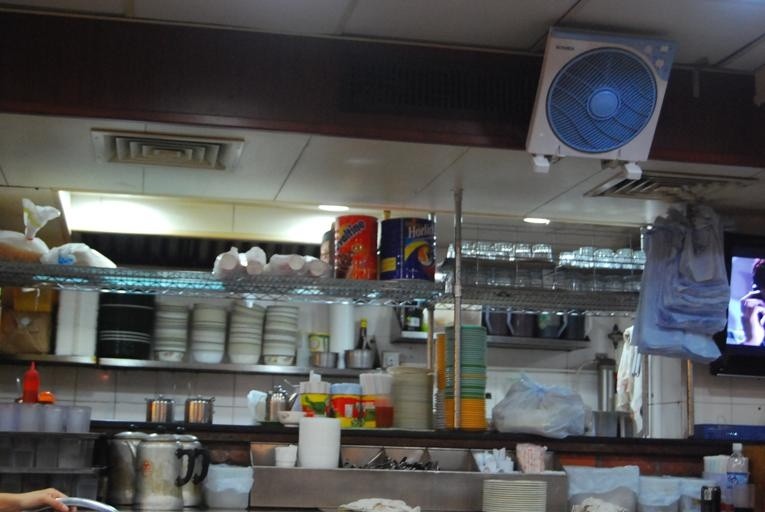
481 478 548 512
296 417 341 470
434 326 492 428
385 364 432 430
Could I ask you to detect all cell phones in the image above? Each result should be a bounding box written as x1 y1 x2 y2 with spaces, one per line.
751 284 765 328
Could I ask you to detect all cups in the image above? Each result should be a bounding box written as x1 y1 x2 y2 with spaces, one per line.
446 237 646 293
272 447 298 467
729 480 757 509
214 246 324 278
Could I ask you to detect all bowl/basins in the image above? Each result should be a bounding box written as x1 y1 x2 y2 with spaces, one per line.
310 352 340 369
274 409 305 429
100 299 153 358
343 349 376 369
153 300 300 369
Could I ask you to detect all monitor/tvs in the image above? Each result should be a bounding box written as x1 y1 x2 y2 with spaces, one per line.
708 230 765 378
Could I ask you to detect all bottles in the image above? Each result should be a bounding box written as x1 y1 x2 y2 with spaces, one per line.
186 395 214 424
23 361 41 405
701 484 721 512
726 443 750 505
594 359 618 411
146 395 175 422
357 317 371 350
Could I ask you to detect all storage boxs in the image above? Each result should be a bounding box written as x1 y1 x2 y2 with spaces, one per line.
13 288 60 311
0 307 55 357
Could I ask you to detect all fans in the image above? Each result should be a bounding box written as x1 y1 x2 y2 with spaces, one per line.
520 23 678 184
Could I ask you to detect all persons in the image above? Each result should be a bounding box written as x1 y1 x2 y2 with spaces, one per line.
739 258 765 347
0 486 78 512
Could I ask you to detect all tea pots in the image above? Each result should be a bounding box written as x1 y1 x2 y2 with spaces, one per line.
97 423 207 512
265 384 300 421
